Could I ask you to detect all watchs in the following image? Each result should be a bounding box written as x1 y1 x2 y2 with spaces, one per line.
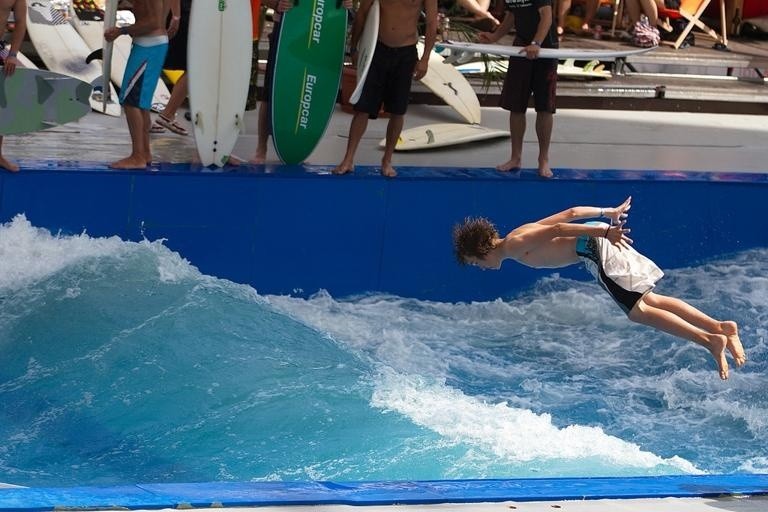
531 40 542 47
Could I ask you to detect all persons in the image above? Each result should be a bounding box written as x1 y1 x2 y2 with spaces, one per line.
622 0 681 40
437 0 501 28
0 0 27 173
248 1 354 167
104 1 172 170
149 0 191 136
330 0 439 178
475 1 559 179
452 194 747 381
557 0 599 35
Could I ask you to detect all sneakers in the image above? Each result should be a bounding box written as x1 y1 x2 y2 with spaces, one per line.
149 120 167 133
156 113 189 136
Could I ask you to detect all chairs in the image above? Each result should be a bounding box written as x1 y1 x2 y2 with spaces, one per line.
553 0 729 49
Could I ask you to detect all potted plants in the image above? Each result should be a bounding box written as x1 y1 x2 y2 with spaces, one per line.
342 0 508 117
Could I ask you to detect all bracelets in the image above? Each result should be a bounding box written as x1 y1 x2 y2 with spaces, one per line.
7 51 17 58
599 207 604 219
604 225 611 238
121 26 128 35
350 47 356 53
171 15 181 21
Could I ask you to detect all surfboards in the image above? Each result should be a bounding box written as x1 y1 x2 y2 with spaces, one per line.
71 0 172 114
162 70 188 99
434 39 659 63
349 1 380 104
0 40 38 69
412 41 481 124
379 124 512 151
0 66 93 135
454 59 612 79
25 0 122 118
271 0 349 165
